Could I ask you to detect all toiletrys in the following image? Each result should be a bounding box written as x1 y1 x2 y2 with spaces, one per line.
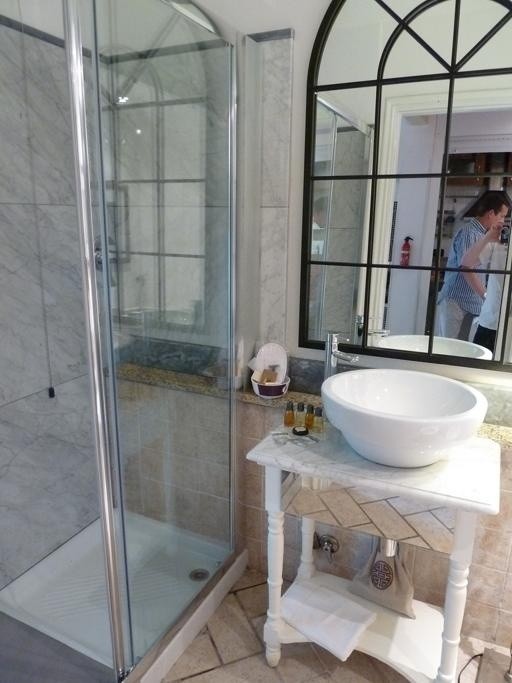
285 400 295 426
305 404 314 429
293 427 308 435
295 402 306 427
314 406 325 433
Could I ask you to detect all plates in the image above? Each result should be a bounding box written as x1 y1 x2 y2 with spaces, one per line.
256 343 288 384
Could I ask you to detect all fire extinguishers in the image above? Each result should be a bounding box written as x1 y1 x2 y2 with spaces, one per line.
400 235 413 265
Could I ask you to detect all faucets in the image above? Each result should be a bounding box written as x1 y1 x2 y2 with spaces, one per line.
323 327 358 375
354 312 391 343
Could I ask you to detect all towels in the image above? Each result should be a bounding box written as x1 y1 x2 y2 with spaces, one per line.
277 579 379 661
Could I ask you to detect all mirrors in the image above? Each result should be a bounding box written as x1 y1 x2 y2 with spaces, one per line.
294 0 512 373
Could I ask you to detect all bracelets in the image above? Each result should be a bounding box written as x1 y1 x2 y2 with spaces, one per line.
481 293 487 300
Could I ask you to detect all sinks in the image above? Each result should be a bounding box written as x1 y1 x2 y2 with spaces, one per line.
319 367 488 471
376 332 492 362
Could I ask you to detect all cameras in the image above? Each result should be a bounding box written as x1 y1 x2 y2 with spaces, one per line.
500 219 511 245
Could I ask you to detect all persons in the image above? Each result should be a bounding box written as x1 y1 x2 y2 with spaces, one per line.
461 219 508 350
436 190 512 341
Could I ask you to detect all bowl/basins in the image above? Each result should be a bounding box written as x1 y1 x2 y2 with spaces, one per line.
250 374 289 400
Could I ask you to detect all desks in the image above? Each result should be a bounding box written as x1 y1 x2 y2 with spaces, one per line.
245 419 502 682
